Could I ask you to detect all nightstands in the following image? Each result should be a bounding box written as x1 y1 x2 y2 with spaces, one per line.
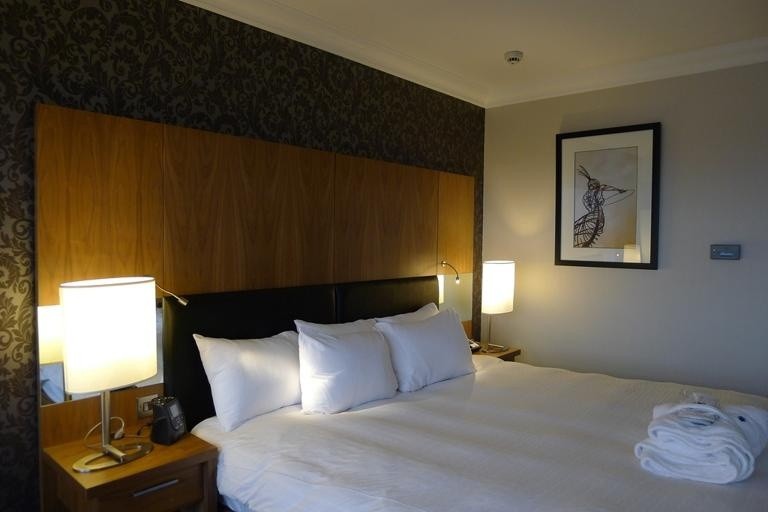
43 421 219 511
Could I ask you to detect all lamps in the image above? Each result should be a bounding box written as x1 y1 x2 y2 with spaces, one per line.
481 259 517 353
57 274 160 475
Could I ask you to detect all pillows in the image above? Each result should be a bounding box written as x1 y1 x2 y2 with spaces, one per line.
192 301 479 434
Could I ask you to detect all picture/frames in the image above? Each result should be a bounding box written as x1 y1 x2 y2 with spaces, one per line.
553 121 663 271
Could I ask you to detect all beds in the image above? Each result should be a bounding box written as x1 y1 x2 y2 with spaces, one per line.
160 274 768 512
